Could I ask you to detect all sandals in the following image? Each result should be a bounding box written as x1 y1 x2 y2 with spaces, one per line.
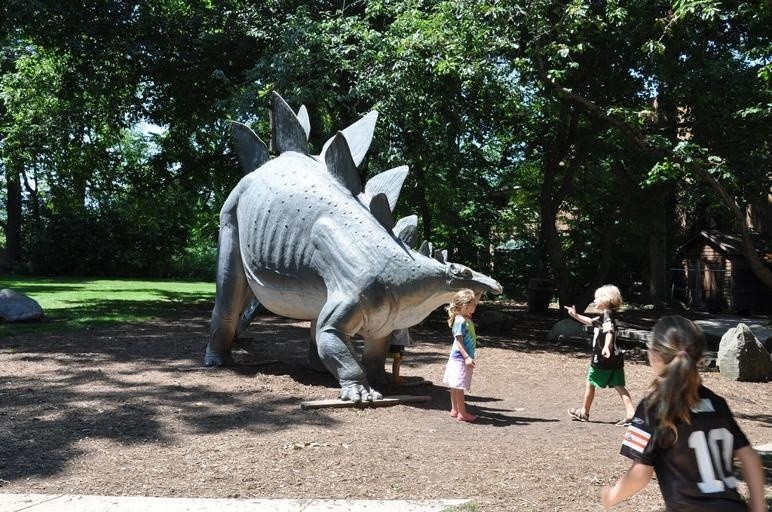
567 407 589 421
615 418 633 426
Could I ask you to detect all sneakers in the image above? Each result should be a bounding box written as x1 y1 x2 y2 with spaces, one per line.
450 408 478 422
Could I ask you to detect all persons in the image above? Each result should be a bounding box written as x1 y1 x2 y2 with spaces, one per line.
446 288 486 422
388 327 413 385
563 284 638 424
599 314 769 512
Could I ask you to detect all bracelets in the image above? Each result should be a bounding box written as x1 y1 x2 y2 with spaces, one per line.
464 356 468 360
